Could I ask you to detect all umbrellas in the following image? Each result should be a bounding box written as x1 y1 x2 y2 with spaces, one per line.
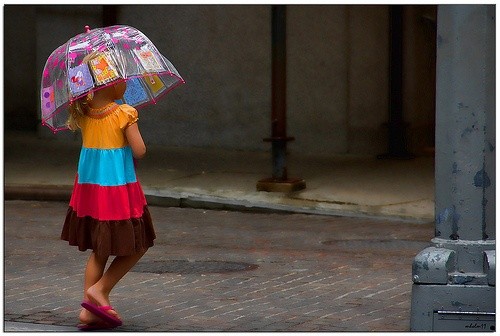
40 23 186 134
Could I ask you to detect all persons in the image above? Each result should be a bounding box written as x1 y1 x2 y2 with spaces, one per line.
63 76 158 333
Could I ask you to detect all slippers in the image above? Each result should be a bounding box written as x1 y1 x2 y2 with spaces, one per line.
78 301 122 329
